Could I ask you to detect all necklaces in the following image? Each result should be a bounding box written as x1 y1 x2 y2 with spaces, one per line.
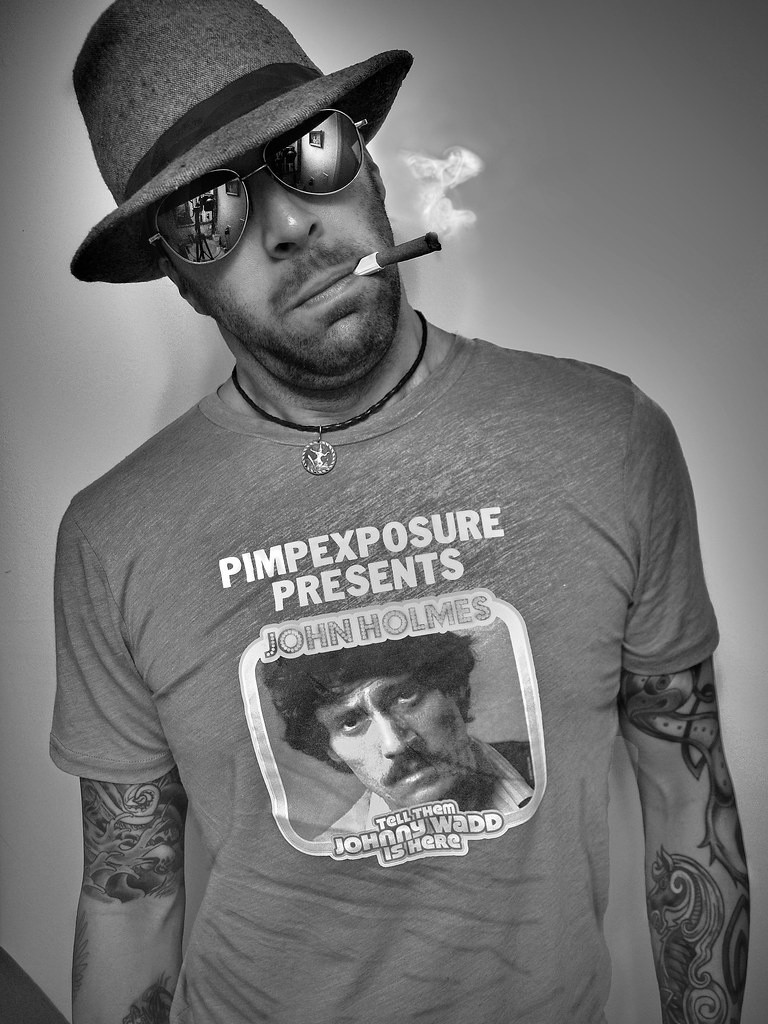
231 309 428 476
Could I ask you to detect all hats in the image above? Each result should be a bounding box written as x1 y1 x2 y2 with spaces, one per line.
69 0 416 287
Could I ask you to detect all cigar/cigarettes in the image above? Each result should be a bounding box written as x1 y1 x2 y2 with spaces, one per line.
354 232 442 276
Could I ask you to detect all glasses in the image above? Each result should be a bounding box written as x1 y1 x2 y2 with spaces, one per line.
142 107 373 267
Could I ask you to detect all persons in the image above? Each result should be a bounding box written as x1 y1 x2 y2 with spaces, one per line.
262 631 536 843
48 0 752 1024
